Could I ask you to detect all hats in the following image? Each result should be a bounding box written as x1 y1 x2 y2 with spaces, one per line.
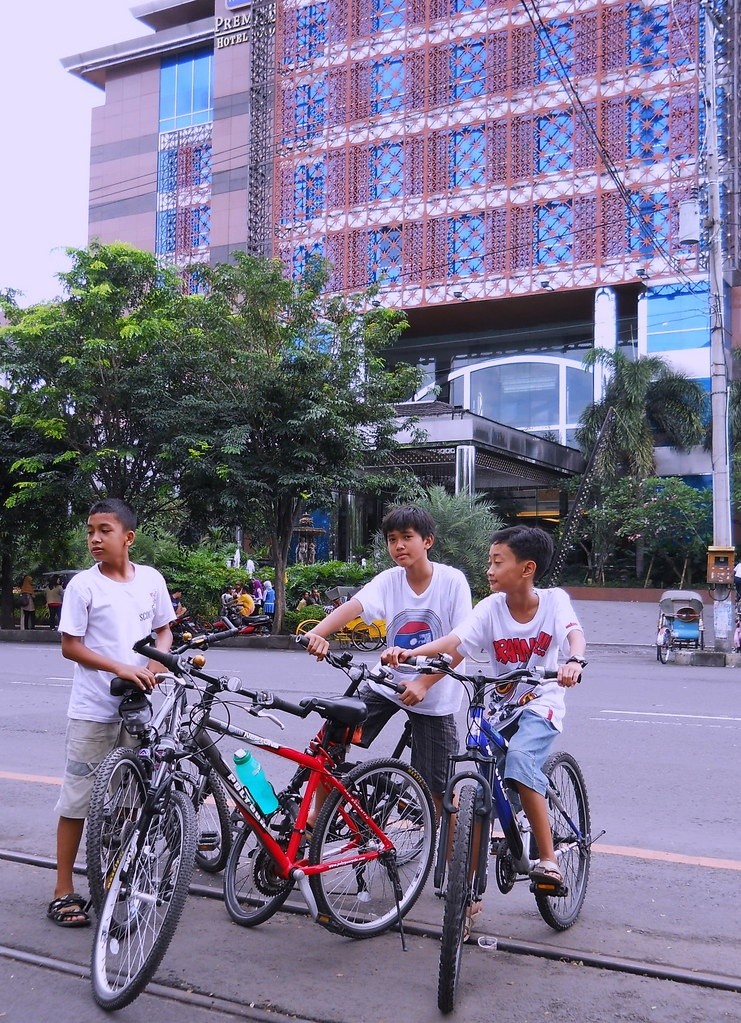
227 585 234 590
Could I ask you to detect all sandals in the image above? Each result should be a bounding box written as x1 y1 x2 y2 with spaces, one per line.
46 892 92 928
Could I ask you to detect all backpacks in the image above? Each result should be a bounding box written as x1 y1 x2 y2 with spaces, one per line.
17 593 31 607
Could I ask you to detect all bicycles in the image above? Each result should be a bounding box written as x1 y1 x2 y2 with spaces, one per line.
381 652 607 1014
84 626 235 941
90 632 437 1012
222 634 439 928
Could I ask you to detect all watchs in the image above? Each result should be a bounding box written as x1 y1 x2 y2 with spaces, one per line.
567 655 587 668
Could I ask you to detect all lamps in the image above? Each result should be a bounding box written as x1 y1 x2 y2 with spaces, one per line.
636 269 650 280
541 281 555 292
454 292 469 301
371 301 386 309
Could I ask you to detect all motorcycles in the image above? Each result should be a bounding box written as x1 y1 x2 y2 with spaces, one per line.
169 605 273 645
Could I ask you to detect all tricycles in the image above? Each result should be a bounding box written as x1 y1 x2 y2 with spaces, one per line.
296 616 386 651
656 590 704 664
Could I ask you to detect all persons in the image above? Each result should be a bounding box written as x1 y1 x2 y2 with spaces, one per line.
21 576 36 630
220 579 275 615
380 524 586 943
47 499 179 927
334 598 344 607
169 588 186 627
45 574 64 631
304 505 482 914
296 588 322 612
295 537 316 564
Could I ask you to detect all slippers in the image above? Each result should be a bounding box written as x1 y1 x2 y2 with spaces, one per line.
440 909 483 941
529 861 564 885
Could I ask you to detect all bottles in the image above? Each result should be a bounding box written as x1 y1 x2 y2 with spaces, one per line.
147 732 179 795
233 748 278 815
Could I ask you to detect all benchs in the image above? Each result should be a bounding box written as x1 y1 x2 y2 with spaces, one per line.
667 618 700 640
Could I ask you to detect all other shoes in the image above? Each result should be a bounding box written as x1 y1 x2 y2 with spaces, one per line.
305 822 313 833
